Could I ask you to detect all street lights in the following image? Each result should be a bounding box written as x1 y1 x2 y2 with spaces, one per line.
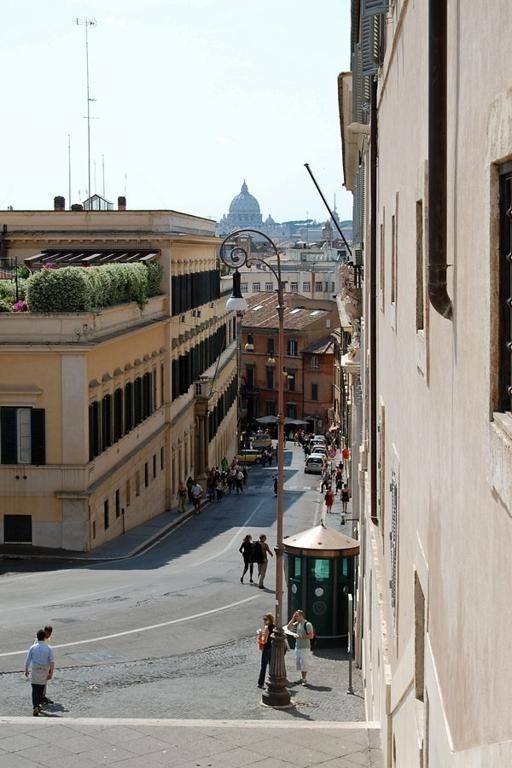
220 229 290 706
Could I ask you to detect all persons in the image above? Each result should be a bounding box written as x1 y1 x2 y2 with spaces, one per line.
295 428 351 513
257 613 276 689
24 625 56 716
287 610 314 686
176 426 286 515
239 534 273 589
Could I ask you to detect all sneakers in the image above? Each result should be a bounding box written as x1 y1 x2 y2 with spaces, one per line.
33 697 53 716
294 679 307 686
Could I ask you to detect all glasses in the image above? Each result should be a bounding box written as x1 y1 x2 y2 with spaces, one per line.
264 618 268 620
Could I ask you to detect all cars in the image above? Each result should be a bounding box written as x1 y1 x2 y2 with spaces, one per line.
252 434 272 449
304 434 328 475
236 448 262 464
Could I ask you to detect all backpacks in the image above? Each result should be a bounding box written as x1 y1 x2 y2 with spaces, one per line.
252 541 261 562
304 621 318 651
